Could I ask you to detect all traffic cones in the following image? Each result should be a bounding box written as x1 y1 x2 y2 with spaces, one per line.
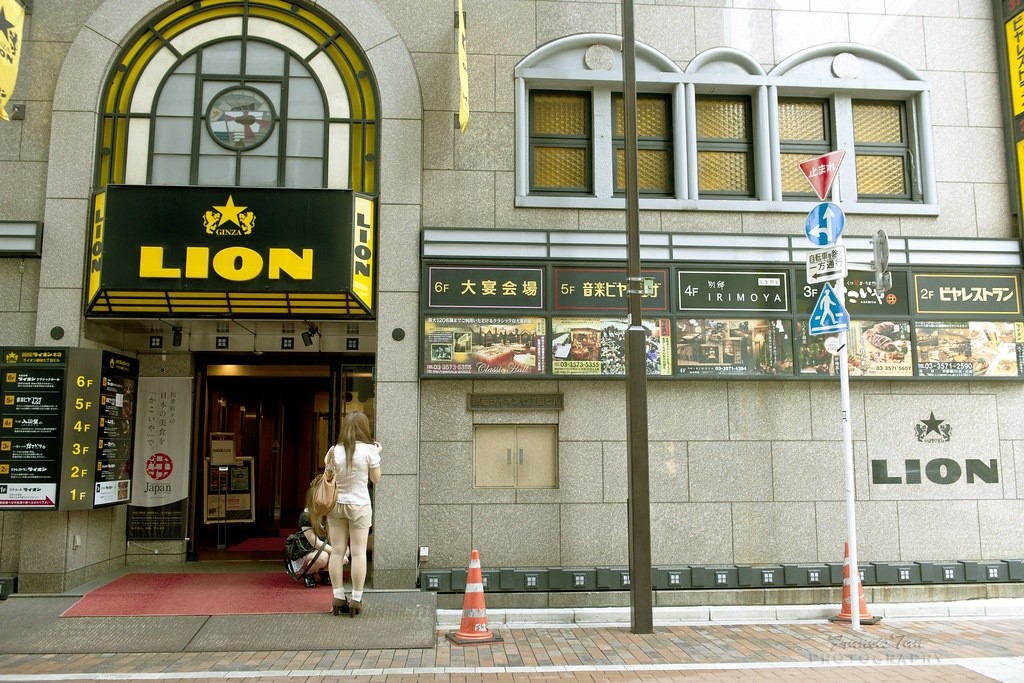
828 539 883 625
443 548 505 647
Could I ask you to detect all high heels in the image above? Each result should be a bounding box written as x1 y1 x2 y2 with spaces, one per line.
332 596 350 618
350 600 363 618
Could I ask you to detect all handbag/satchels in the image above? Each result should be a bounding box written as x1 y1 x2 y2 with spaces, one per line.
306 445 338 517
284 527 316 561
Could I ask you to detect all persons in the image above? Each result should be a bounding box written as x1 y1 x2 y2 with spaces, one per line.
282 505 350 588
322 412 384 616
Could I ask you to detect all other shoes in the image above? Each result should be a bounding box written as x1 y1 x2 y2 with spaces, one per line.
304 574 316 588
318 571 332 585
